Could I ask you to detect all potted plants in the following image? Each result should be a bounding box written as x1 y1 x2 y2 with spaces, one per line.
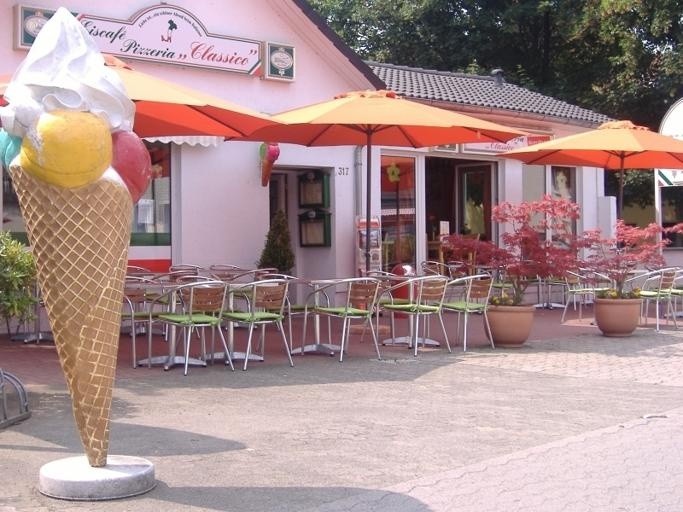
569 220 683 338
436 194 584 348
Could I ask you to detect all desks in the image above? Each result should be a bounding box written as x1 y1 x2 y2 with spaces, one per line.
126 279 208 370
188 279 267 363
381 276 439 348
290 276 347 356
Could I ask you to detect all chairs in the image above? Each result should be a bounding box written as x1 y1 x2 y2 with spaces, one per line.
211 264 245 332
420 275 495 353
220 280 296 372
228 266 280 282
147 281 238 376
257 274 331 355
356 275 452 357
168 262 222 339
122 276 164 369
644 268 683 334
577 267 616 320
125 264 170 343
300 277 385 361
560 269 596 325
624 267 678 334
447 257 465 267
422 258 451 280
